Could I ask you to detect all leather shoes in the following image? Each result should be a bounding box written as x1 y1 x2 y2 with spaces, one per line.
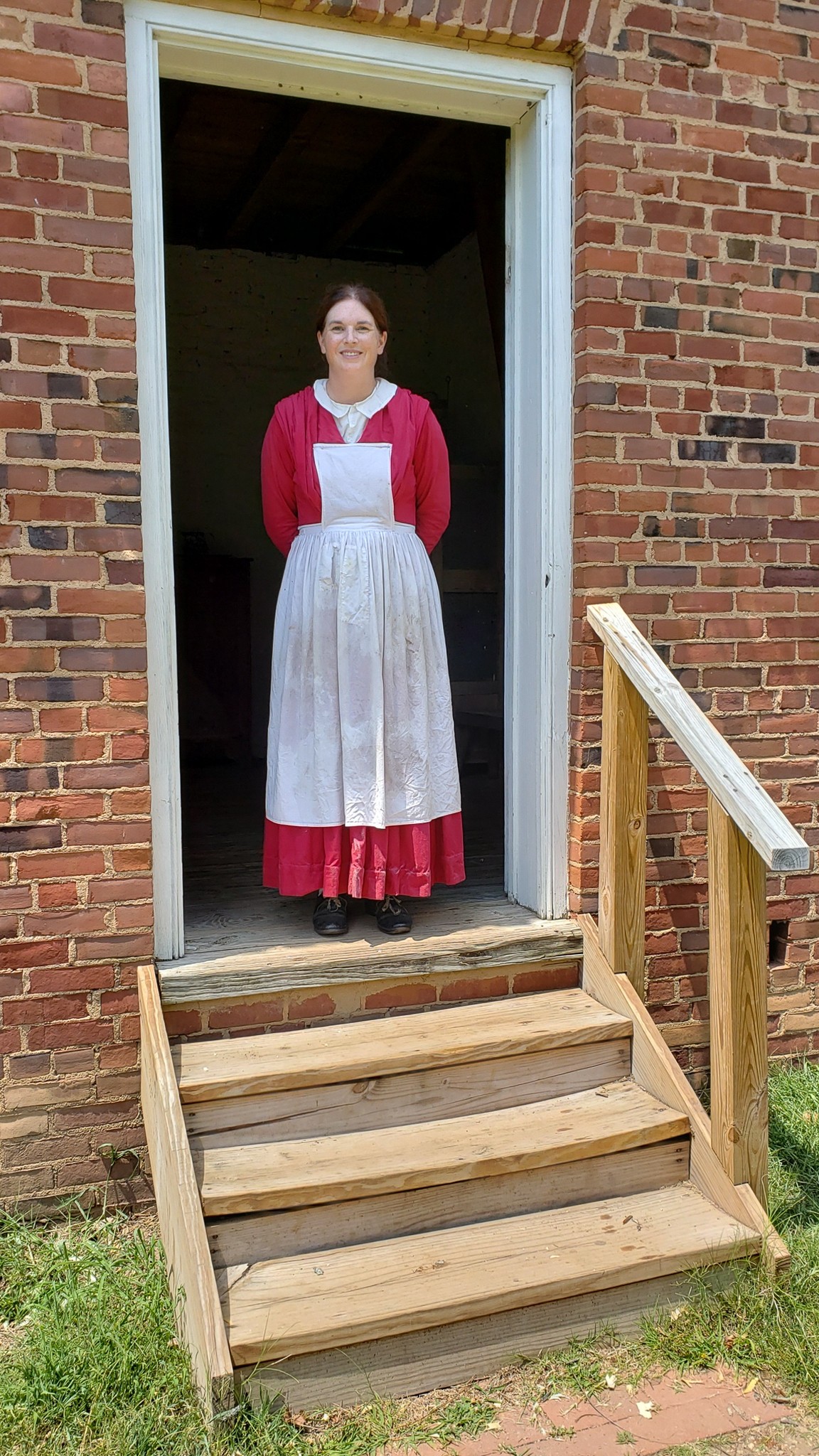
364 895 411 933
312 892 349 933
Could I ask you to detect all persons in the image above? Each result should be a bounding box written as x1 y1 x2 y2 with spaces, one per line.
261 281 468 935
